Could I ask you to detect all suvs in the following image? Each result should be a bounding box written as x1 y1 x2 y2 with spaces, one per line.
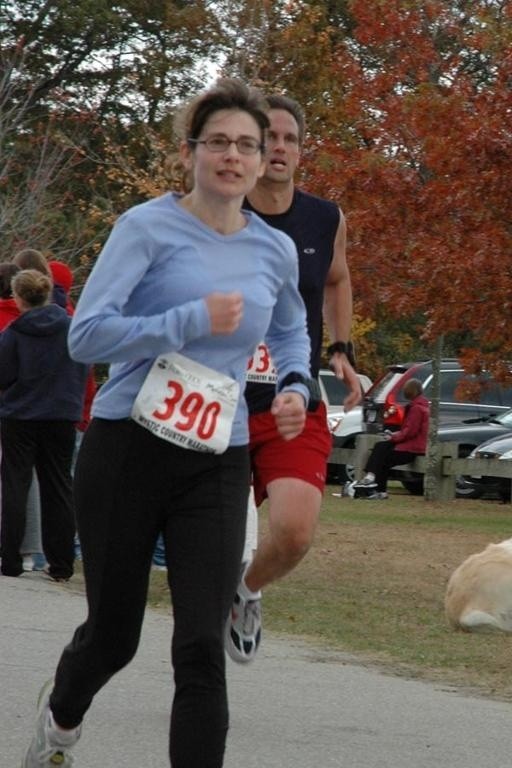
363 355 512 500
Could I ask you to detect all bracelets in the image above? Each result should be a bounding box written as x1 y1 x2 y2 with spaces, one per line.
279 370 322 413
323 341 359 366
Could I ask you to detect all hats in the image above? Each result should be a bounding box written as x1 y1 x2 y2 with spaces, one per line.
48 261 72 292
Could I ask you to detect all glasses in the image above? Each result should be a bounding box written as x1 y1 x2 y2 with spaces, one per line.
187 133 265 155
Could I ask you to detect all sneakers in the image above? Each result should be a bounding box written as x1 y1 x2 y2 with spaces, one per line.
224 561 263 662
19 676 83 768
353 476 388 499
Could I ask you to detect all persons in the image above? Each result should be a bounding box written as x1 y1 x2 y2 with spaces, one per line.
1 262 24 332
0 267 90 584
348 377 432 500
220 93 365 667
20 259 96 578
13 247 53 275
19 75 323 768
